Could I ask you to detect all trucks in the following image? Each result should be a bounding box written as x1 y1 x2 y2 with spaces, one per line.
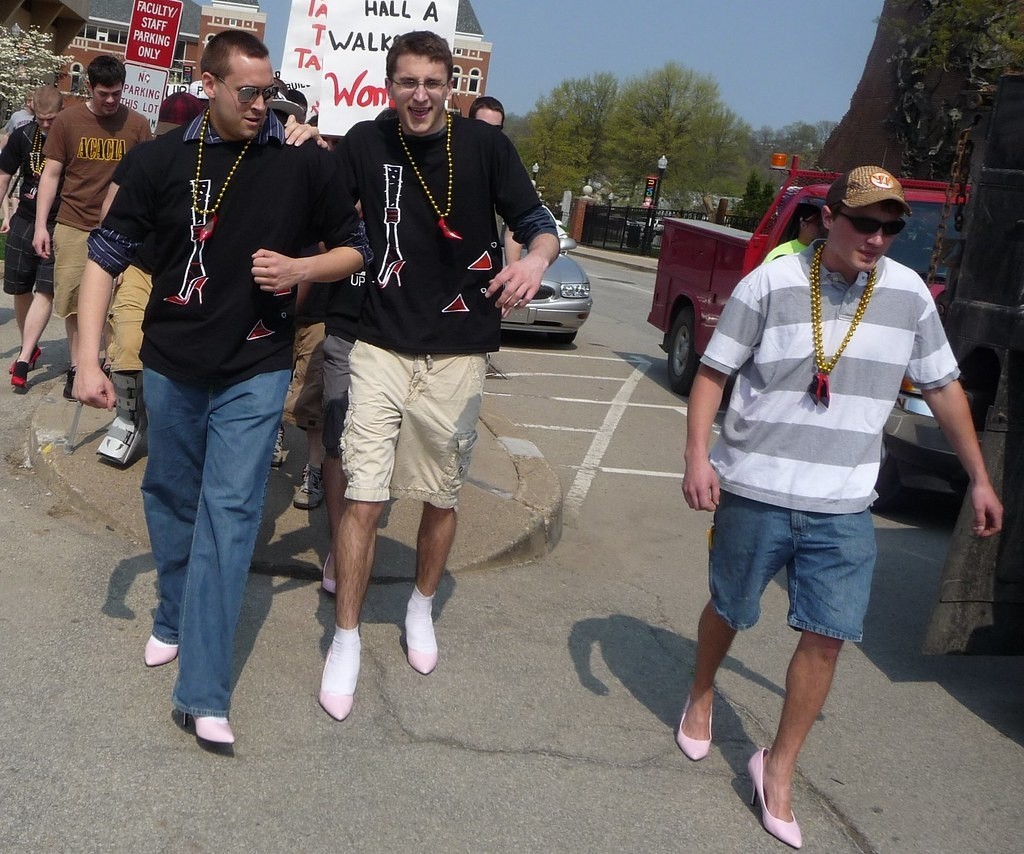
648 154 968 416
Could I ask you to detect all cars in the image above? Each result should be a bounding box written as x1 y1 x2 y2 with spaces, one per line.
500 203 593 344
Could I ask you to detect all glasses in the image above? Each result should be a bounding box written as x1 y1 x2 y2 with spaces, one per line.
390 76 450 94
835 211 906 236
213 74 279 107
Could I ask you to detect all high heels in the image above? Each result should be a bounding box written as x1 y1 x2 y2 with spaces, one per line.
405 603 439 676
676 692 712 761
322 549 338 594
320 642 357 720
181 709 235 747
9 346 41 387
143 635 180 667
747 748 803 849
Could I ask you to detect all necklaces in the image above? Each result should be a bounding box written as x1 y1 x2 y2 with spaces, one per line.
399 111 463 242
25 127 46 185
807 242 877 408
192 109 253 242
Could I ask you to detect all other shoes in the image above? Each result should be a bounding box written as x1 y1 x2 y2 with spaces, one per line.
63 368 79 401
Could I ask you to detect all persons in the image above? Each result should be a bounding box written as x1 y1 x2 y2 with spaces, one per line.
284 31 560 722
0 85 63 390
678 165 1003 849
31 55 210 464
265 77 524 594
0 91 37 234
761 208 825 265
71 30 374 744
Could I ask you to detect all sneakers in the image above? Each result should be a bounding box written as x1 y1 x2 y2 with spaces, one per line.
270 425 285 467
293 463 324 510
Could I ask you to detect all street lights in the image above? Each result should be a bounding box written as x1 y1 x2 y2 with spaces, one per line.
640 155 669 259
530 162 540 189
602 192 614 250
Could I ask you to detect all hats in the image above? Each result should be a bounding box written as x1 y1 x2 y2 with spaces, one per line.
154 92 202 135
825 165 912 216
191 80 210 99
266 92 304 120
